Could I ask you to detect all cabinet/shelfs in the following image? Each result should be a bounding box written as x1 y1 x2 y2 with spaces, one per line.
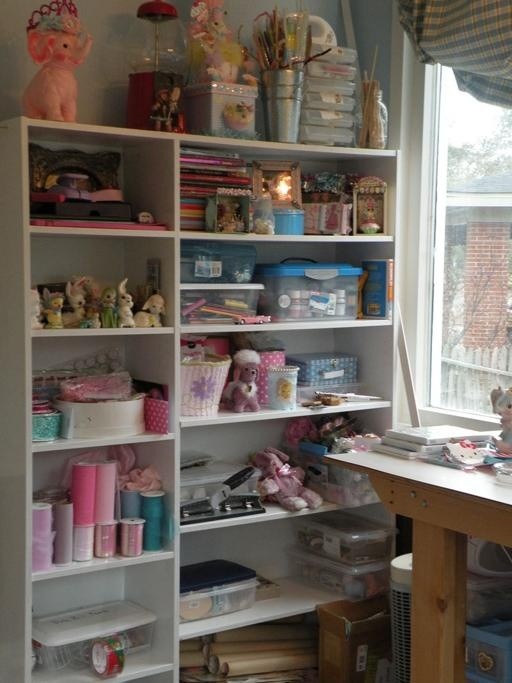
2 116 403 683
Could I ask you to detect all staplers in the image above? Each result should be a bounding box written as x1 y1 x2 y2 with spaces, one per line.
180 467 266 526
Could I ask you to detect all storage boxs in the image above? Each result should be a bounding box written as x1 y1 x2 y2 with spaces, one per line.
288 443 379 509
32 600 159 673
287 551 393 603
181 241 255 282
295 512 399 566
286 353 361 383
178 459 263 506
464 623 511 683
315 599 389 681
179 578 260 623
183 284 264 323
259 258 364 322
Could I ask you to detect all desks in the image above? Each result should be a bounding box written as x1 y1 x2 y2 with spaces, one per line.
324 432 511 683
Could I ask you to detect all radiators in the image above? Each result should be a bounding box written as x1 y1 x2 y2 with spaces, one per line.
389 553 414 683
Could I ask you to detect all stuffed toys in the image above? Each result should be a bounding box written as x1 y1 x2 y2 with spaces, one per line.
223 349 263 413
21 0 94 123
491 385 512 456
183 1 247 85
30 274 168 330
147 83 185 132
247 444 325 512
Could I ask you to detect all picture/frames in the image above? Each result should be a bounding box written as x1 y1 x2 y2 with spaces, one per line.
249 159 301 209
351 175 389 235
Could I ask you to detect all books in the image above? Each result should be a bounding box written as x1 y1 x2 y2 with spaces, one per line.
180 147 252 231
369 424 490 461
